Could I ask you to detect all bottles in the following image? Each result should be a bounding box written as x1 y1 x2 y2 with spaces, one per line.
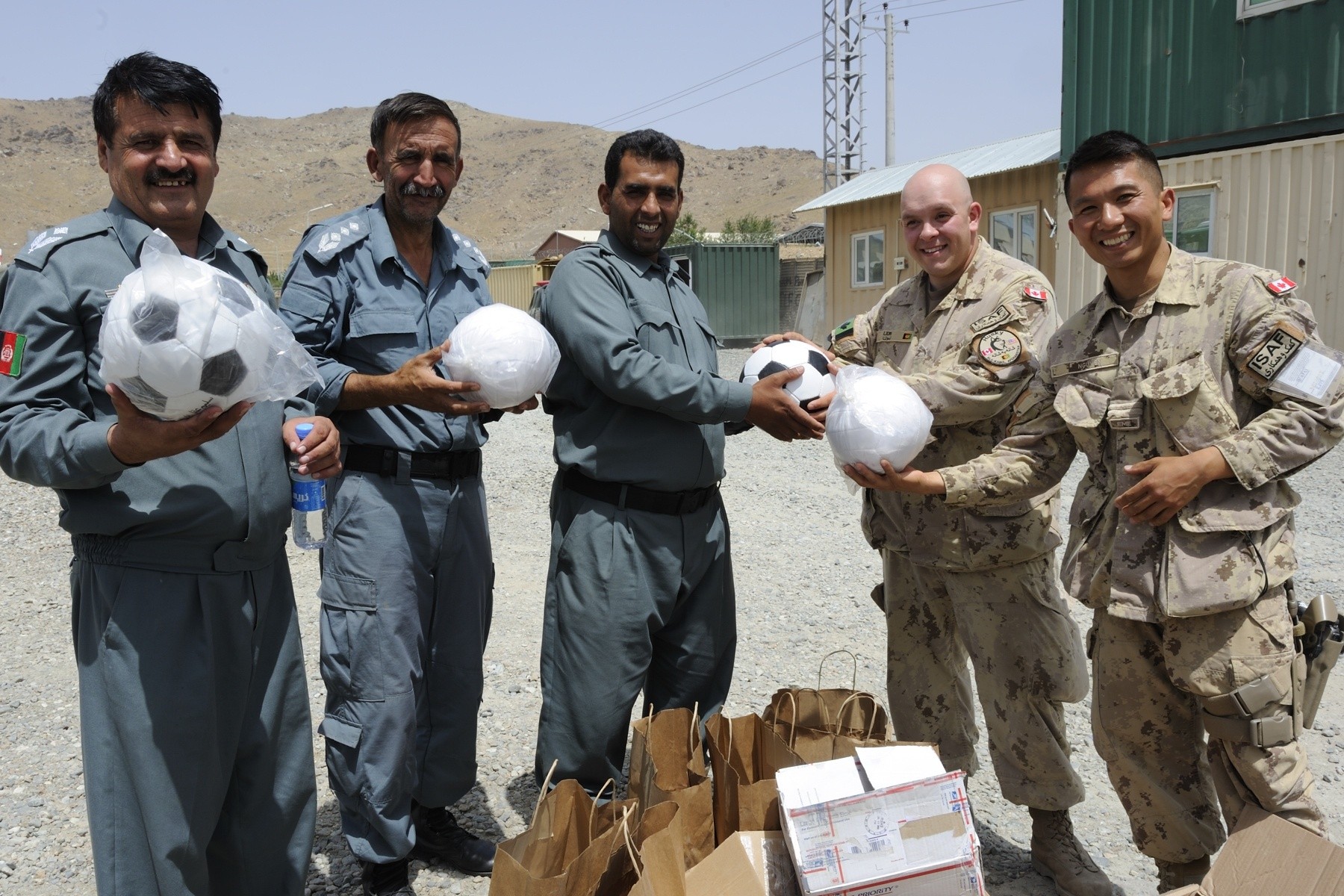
289 423 329 550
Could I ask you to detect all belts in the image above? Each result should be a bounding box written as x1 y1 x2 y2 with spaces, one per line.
556 465 718 516
343 444 482 480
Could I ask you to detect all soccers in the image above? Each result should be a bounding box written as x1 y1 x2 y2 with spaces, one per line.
739 339 837 414
826 372 927 474
102 257 273 421
444 302 553 410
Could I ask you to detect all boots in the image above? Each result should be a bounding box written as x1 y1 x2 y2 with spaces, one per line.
1156 854 1211 896
1028 805 1112 896
405 798 497 876
357 854 417 896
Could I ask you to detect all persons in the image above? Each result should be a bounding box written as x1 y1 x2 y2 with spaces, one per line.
276 92 538 896
750 163 1114 896
528 128 828 809
0 48 342 896
838 129 1344 896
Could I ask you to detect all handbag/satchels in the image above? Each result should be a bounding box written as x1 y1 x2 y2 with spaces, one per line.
762 649 941 766
628 700 716 875
704 702 811 846
489 758 639 896
586 799 689 896
